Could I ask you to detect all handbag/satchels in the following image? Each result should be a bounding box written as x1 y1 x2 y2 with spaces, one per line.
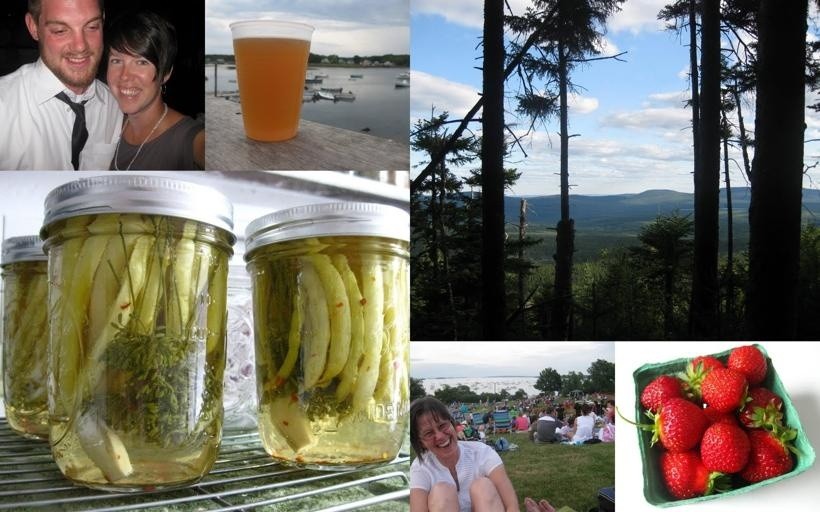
495 437 509 451
584 436 601 444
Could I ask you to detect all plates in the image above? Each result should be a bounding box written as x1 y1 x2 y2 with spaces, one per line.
633 345 815 508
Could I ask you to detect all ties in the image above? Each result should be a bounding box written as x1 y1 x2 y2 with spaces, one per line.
55 91 88 169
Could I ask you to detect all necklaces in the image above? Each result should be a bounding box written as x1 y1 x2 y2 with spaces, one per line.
114 102 168 171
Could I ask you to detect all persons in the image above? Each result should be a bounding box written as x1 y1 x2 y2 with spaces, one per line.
480 397 528 434
0 0 124 170
523 497 575 512
410 397 519 512
105 11 204 170
528 396 615 444
448 398 479 441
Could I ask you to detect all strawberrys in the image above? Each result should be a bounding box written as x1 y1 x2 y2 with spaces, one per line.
701 405 739 433
662 447 709 499
742 429 793 482
701 423 752 473
686 356 725 375
654 396 702 452
737 387 781 428
728 346 765 385
701 363 748 413
640 375 689 410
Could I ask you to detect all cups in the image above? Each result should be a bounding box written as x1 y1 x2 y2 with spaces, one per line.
229 20 315 141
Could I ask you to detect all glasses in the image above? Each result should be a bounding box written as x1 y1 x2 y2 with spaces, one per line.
419 420 451 442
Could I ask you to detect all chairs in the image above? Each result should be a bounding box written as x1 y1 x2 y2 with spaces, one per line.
472 412 483 426
493 411 513 438
